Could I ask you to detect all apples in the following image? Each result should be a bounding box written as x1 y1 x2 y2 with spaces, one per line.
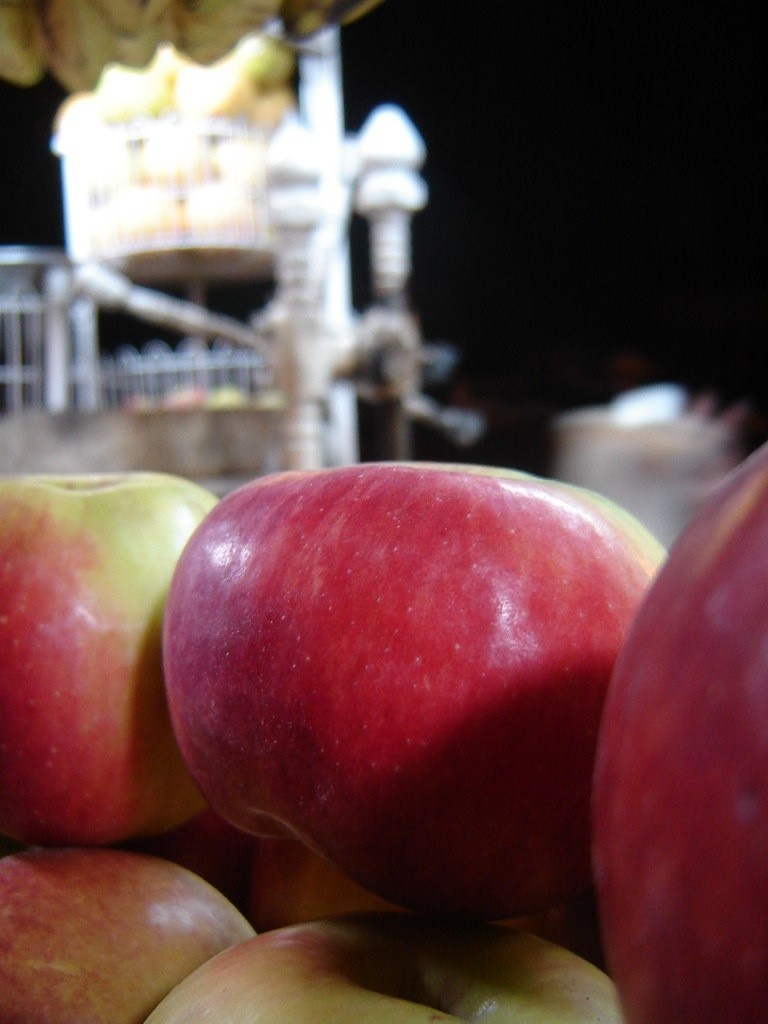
0 442 768 1024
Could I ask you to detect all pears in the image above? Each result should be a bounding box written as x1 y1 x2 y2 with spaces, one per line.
47 29 293 281
